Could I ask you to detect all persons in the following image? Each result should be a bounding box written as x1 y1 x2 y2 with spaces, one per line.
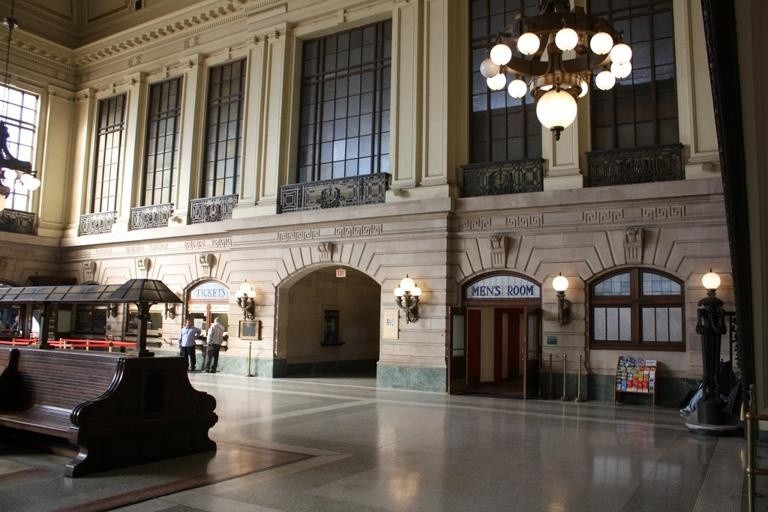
10 315 19 335
178 319 202 371
201 316 225 373
679 370 737 415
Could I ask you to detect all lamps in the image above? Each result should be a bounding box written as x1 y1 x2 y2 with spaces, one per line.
701 269 722 298
394 273 422 324
478 1 634 142
552 272 572 328
235 279 256 321
1 1 42 212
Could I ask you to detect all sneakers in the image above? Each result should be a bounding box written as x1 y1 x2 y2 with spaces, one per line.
680 408 689 413
190 368 216 373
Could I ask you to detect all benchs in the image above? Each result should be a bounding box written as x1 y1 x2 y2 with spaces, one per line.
0 348 137 479
